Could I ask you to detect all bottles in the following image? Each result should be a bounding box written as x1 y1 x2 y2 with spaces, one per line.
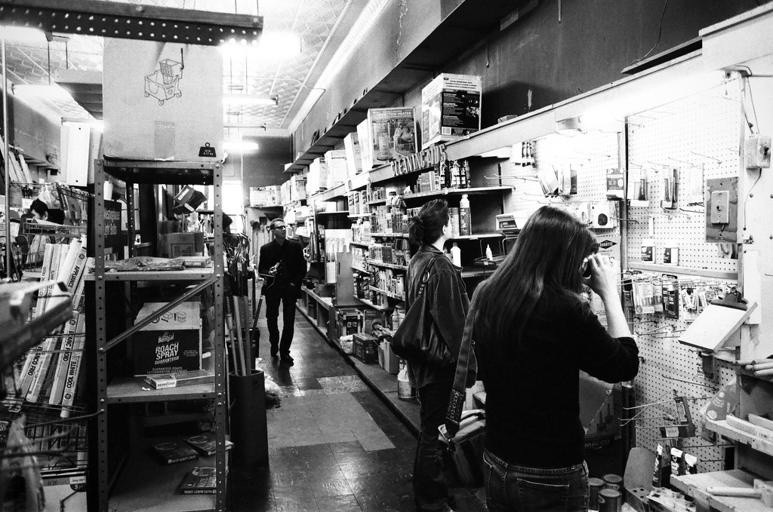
448 240 461 268
348 206 422 334
585 472 623 511
449 194 473 237
448 161 471 191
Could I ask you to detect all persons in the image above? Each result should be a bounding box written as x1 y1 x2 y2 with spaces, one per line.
472 206 640 512
258 218 306 363
405 199 478 512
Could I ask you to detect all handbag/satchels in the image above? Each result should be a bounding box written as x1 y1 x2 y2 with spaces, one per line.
389 277 481 389
436 407 494 490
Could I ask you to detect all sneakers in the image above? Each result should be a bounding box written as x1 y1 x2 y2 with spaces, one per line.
280 350 295 363
270 340 279 357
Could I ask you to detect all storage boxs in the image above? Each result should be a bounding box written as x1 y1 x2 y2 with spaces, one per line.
248 73 483 206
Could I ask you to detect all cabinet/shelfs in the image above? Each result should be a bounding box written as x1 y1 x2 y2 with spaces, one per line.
93 157 227 512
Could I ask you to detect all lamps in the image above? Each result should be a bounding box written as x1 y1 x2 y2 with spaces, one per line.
11 83 64 100
224 120 268 136
224 93 279 107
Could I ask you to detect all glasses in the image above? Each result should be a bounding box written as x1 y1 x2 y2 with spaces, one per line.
272 225 287 230
